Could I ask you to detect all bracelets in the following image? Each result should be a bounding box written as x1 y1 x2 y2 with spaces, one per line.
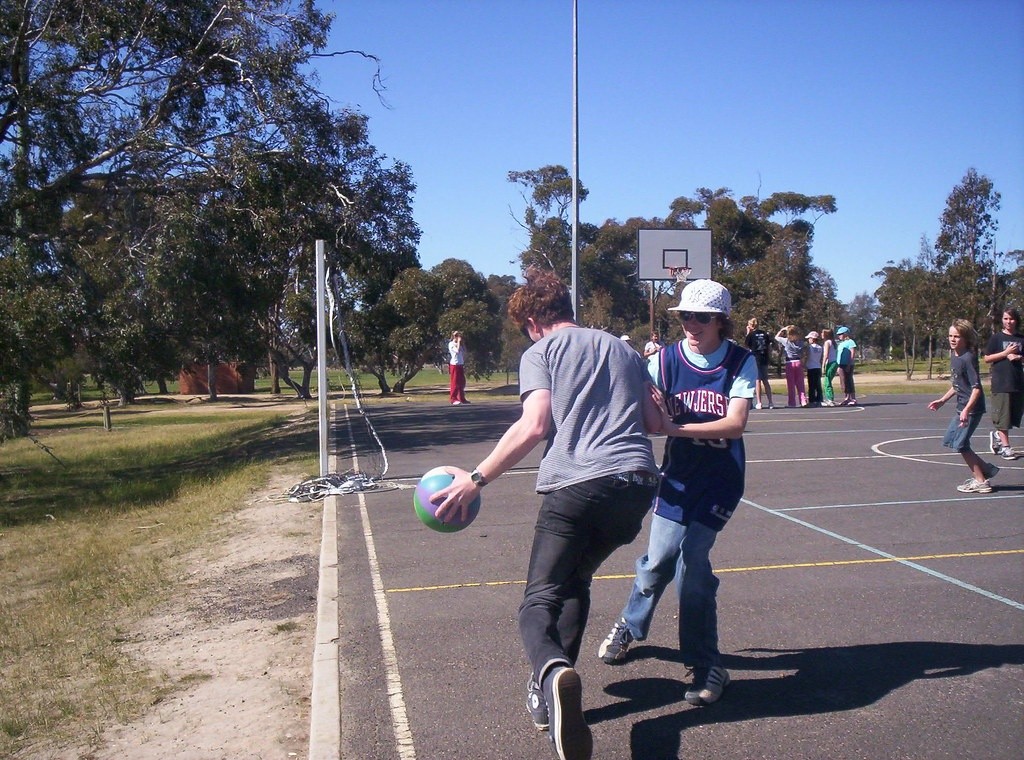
1019 355 1022 360
940 398 946 403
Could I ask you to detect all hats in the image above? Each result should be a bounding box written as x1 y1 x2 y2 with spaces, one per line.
837 327 850 335
668 279 731 317
805 331 819 338
620 335 630 340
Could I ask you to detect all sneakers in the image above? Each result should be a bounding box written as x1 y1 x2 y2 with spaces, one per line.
1001 447 1015 459
599 618 635 665
990 430 1001 455
526 673 549 730
684 660 731 705
542 663 593 760
956 478 992 493
984 463 999 479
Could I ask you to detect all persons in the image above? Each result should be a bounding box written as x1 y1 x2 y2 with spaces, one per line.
804 331 823 408
598 278 759 705
836 327 857 407
774 324 807 408
448 331 471 406
821 328 838 407
427 273 661 760
620 333 632 345
744 315 774 409
983 311 1024 460
643 330 663 434
928 319 999 494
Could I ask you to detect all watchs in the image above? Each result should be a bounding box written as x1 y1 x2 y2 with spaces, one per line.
470 468 488 488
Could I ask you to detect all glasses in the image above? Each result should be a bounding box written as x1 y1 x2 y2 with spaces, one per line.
521 320 538 338
675 311 711 323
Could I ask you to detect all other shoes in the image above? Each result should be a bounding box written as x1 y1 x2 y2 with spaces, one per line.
847 399 856 406
462 400 470 404
839 398 850 405
755 403 762 409
769 403 774 409
452 401 461 404
821 400 836 406
803 402 821 407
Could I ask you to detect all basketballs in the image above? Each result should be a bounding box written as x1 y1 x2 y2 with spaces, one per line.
412 465 480 534
